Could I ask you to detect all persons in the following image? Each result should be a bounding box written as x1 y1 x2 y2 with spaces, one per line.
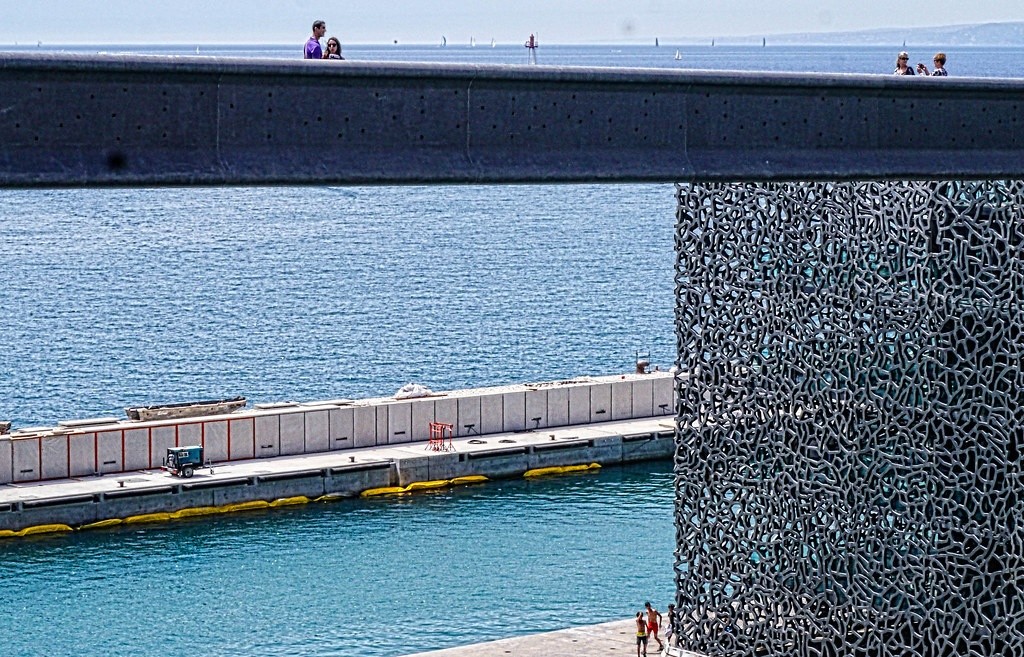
917 53 947 76
635 602 676 657
893 52 916 75
304 21 346 60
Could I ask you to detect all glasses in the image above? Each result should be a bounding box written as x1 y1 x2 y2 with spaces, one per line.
328 44 337 47
900 57 909 60
934 60 937 62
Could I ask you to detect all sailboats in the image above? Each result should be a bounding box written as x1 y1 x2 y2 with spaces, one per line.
674 47 682 60
470 37 477 47
436 36 446 48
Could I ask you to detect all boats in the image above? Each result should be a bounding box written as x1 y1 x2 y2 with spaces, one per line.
124 393 247 422
